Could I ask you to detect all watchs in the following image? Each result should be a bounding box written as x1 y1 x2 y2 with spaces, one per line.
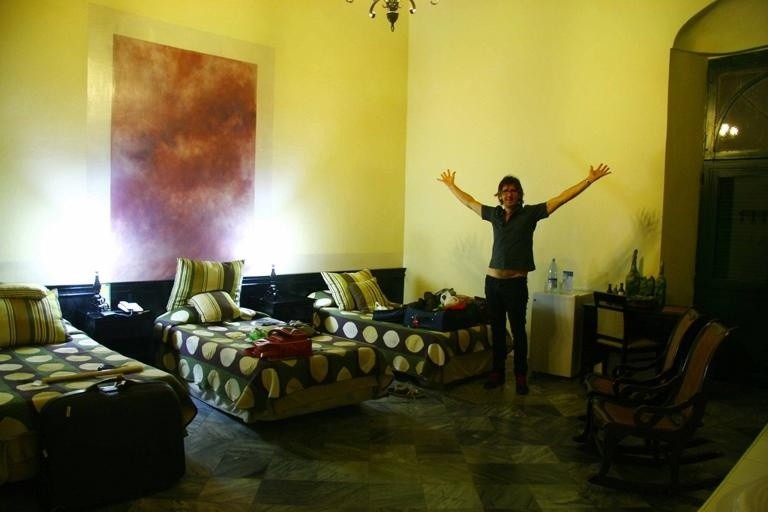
585 178 591 184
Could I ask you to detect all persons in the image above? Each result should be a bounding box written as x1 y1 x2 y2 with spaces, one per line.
436 163 612 396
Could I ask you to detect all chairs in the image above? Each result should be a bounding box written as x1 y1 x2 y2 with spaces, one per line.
591 290 662 377
551 307 705 444
572 317 738 493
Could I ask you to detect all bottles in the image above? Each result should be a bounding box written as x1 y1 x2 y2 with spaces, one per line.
547 257 558 292
617 283 625 296
606 283 612 306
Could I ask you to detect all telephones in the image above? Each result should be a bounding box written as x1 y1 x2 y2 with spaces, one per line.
117 300 145 314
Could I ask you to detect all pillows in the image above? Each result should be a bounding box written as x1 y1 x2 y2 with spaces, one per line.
311 298 336 308
167 258 245 310
307 289 331 299
237 308 270 320
0 284 49 299
153 307 200 325
348 277 392 312
319 268 374 310
0 296 73 345
189 291 241 323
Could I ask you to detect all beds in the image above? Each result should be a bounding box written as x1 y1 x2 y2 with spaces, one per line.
153 296 394 423
320 275 511 399
0 289 200 512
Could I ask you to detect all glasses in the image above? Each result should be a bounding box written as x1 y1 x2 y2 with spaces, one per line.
501 189 519 196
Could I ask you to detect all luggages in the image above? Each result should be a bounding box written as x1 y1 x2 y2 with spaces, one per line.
372 298 474 331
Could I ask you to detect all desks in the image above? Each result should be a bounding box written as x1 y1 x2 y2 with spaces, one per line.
581 302 694 375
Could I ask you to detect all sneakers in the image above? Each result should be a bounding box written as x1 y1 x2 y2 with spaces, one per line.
515 373 529 395
482 371 506 390
394 382 426 399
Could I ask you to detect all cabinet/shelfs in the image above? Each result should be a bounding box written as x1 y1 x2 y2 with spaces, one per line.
527 288 593 382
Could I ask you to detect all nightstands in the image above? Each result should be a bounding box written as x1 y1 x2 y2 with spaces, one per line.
71 303 150 356
255 289 319 329
